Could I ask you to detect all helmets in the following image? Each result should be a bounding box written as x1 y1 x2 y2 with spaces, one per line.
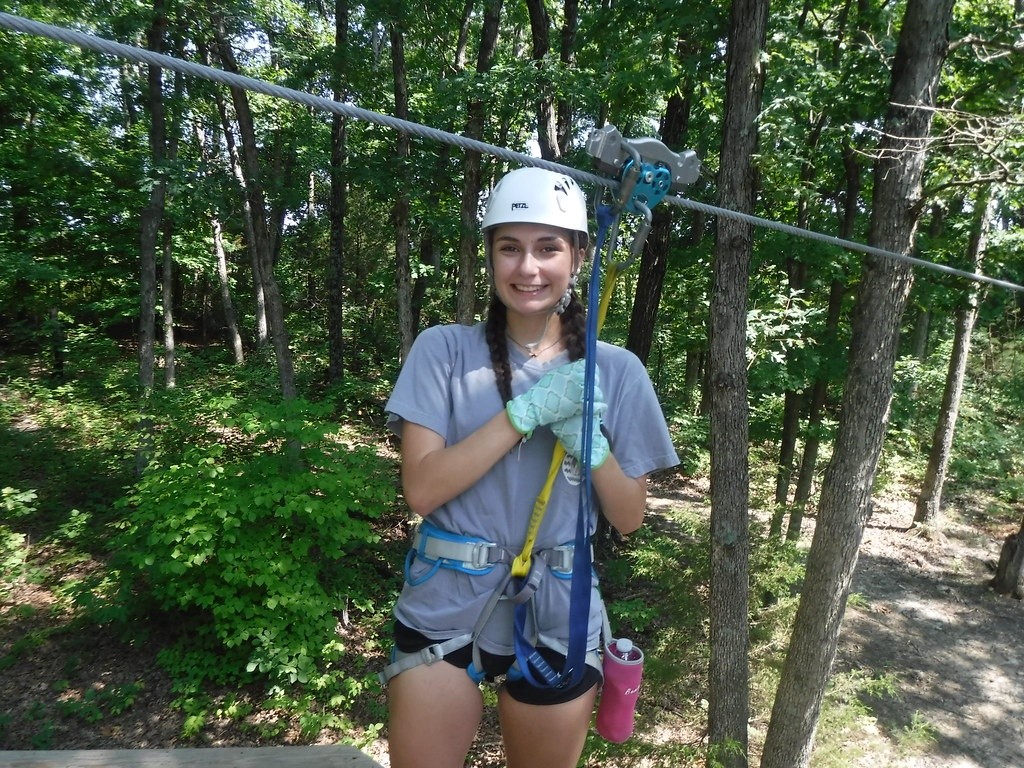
482 167 590 251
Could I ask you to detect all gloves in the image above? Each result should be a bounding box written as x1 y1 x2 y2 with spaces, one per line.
548 415 610 471
505 357 608 442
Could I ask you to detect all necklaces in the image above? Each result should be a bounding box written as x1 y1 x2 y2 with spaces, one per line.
506 334 565 357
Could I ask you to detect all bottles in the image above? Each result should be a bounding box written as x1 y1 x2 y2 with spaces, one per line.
613 638 636 662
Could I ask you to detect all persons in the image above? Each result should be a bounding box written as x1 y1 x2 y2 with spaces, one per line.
386 167 682 768
